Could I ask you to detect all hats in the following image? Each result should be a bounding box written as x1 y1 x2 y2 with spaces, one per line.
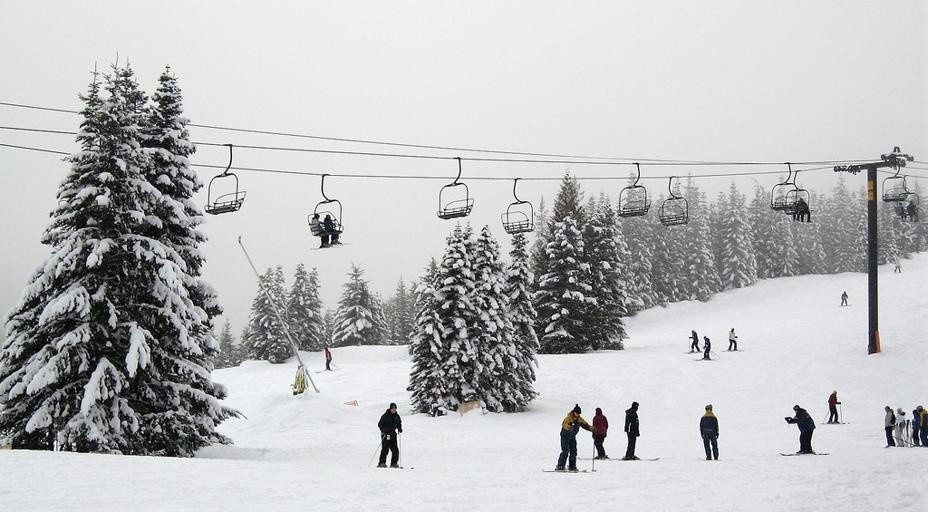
390 403 396 408
575 404 581 413
632 402 638 409
706 405 712 410
794 405 800 411
596 408 601 412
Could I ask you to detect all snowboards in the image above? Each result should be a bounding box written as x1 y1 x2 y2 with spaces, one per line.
578 456 657 461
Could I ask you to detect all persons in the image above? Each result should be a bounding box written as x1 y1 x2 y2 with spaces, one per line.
699 403 721 461
688 330 700 354
376 402 404 468
621 400 640 461
909 409 920 447
555 405 596 470
898 201 907 222
323 346 332 371
798 198 812 223
323 214 341 245
915 405 928 447
592 407 609 460
892 258 901 274
310 213 329 247
783 405 816 455
907 200 919 222
727 328 738 351
894 408 906 448
839 291 848 306
702 336 711 360
826 388 842 425
882 405 895 449
791 198 799 223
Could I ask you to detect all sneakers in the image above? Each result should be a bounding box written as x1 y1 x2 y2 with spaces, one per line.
391 463 399 467
556 465 578 471
379 463 386 467
596 455 607 459
625 455 638 460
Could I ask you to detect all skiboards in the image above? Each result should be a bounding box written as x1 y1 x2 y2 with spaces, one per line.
685 347 741 362
370 458 413 469
541 469 596 474
779 452 829 456
